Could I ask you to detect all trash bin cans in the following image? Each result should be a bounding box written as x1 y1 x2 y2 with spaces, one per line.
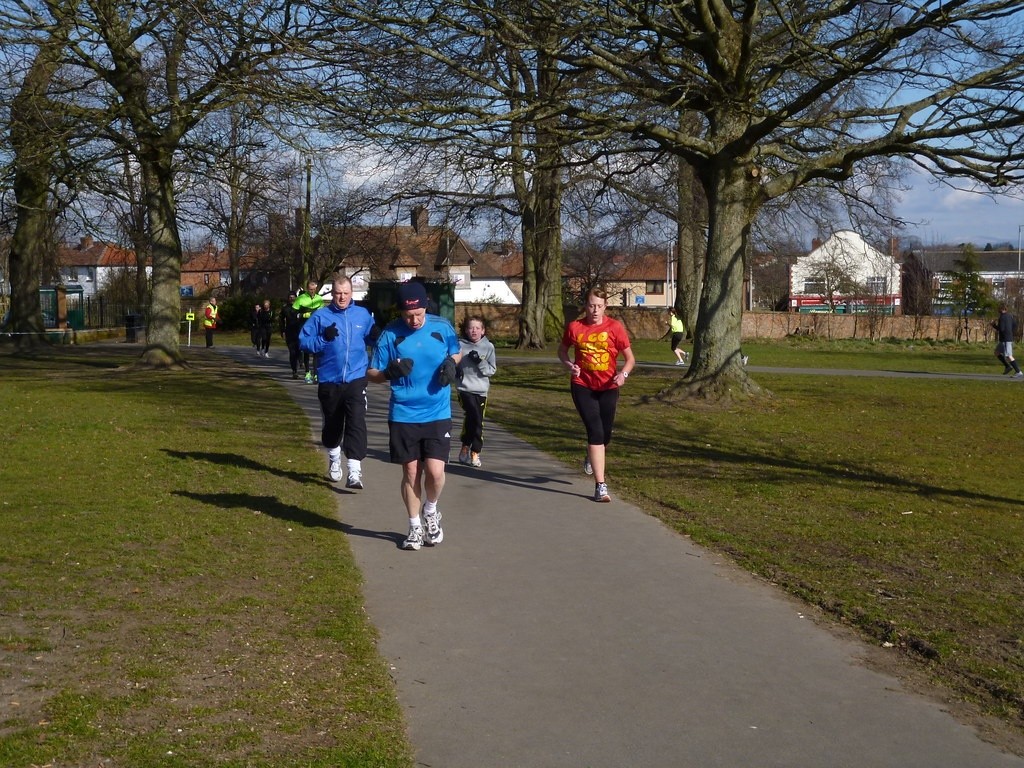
125 315 147 343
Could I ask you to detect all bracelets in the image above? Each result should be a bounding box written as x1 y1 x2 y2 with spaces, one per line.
621 371 628 377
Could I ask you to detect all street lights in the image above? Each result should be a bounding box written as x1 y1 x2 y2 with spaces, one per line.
890 216 905 317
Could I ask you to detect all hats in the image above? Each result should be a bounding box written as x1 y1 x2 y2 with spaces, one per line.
396 282 428 310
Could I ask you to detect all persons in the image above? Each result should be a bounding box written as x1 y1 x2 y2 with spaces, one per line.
204 296 221 349
665 306 689 365
455 315 496 467
990 303 1023 378
279 280 324 384
366 282 461 551
250 300 273 359
558 287 635 502
299 276 382 489
741 354 749 366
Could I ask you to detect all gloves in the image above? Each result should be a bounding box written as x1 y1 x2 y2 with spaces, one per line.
384 359 414 380
467 350 482 364
455 367 464 381
323 322 339 342
369 325 380 342
438 357 457 387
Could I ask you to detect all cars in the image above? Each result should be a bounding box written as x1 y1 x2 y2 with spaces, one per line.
40 309 70 329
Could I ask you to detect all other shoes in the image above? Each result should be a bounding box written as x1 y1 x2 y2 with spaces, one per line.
303 371 313 384
684 353 689 359
1002 369 1010 374
675 361 684 365
300 364 304 369
313 375 318 382
743 356 749 366
206 346 214 348
293 375 299 379
264 354 270 359
1010 372 1022 379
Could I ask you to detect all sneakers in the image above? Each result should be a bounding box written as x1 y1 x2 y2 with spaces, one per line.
459 443 470 463
595 484 611 502
346 473 363 489
471 451 481 467
584 457 593 475
328 453 343 481
402 526 425 550
422 504 443 544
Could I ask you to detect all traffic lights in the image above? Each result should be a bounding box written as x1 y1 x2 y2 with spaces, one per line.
620 288 627 308
